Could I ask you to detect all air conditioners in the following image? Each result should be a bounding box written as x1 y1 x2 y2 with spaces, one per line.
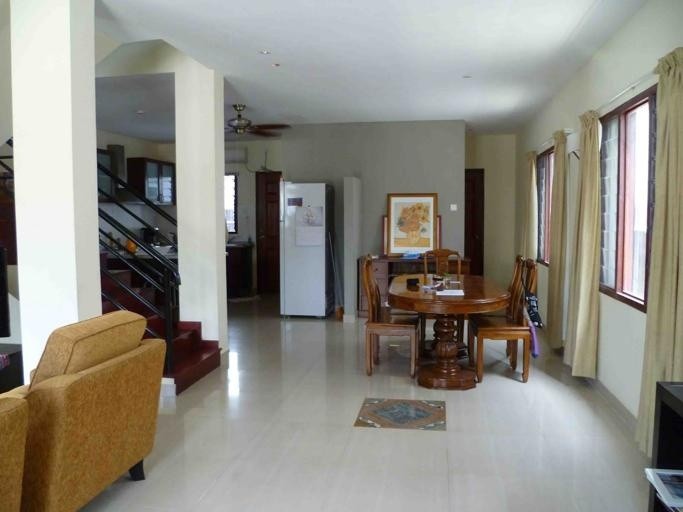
225 148 247 163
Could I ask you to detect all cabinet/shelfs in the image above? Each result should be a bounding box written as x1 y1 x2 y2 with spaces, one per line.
647 380 683 512
125 157 175 206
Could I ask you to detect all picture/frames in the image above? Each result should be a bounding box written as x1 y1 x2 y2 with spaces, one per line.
383 215 441 255
386 193 437 257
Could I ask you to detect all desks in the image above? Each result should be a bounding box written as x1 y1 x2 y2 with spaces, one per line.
359 254 471 318
226 241 255 297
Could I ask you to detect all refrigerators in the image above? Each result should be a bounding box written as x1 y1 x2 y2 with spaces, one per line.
279 179 335 319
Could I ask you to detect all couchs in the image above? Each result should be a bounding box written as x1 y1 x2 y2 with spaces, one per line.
0 397 30 512
1 308 166 511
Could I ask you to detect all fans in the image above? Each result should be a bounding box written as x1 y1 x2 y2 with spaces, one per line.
225 103 292 138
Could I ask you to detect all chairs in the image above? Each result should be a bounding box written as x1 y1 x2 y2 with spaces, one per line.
367 252 418 315
467 258 538 383
421 248 464 343
467 255 524 356
362 257 419 377
